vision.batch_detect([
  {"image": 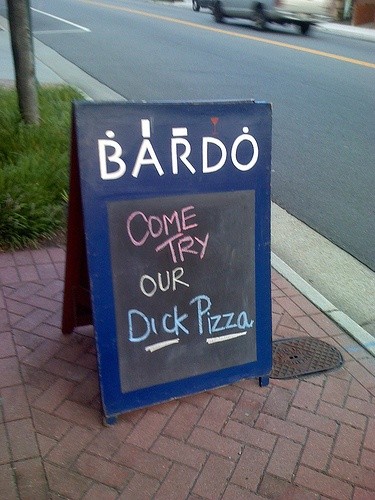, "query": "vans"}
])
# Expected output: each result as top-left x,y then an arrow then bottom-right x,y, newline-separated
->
190,1 -> 331,36
